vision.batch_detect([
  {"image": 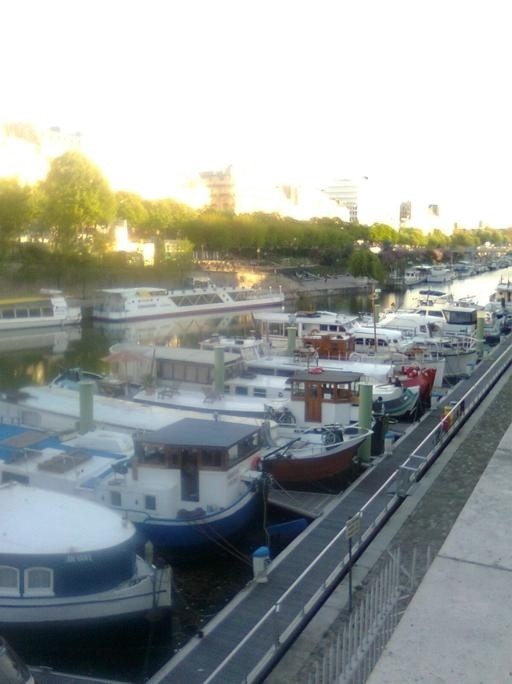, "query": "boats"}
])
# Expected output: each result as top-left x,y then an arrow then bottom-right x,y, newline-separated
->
98,293 -> 486,426
404,256 -> 512,286
92,285 -> 286,332
486,273 -> 512,347
1,382 -> 273,549
0,289 -> 82,330
12,387 -> 373,482
0,479 -> 171,636
